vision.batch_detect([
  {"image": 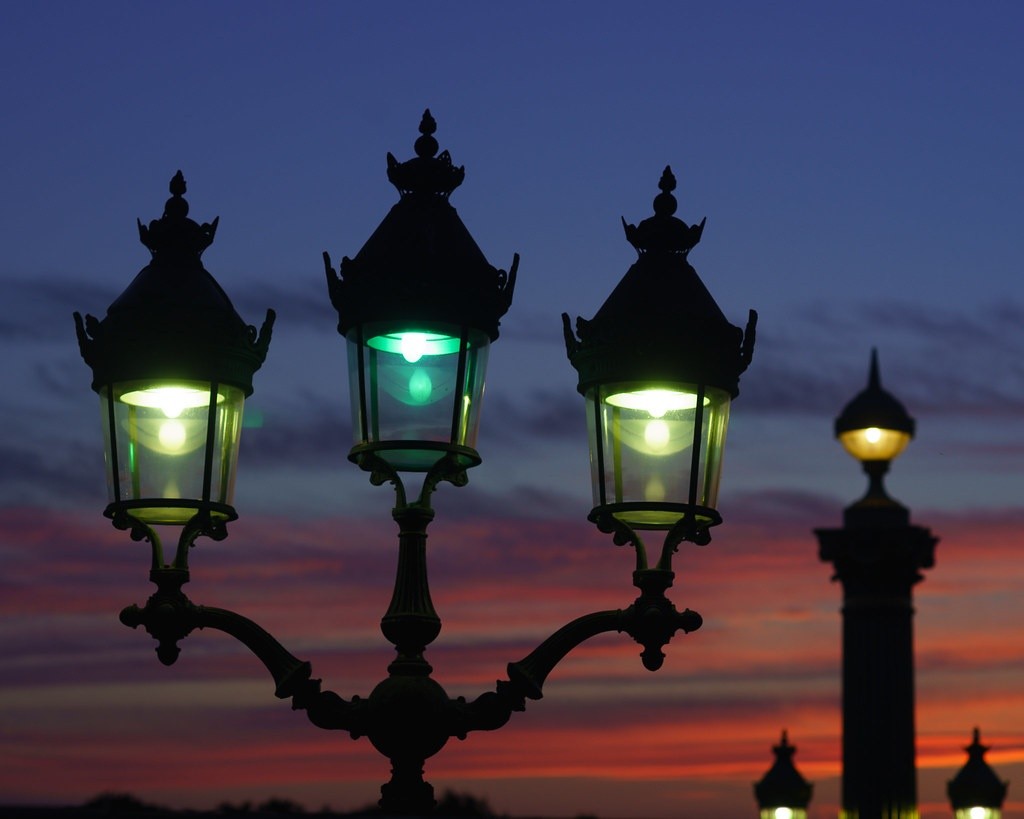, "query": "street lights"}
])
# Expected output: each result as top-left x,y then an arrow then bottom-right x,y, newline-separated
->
753,345 -> 1011,819
74,103 -> 757,819
